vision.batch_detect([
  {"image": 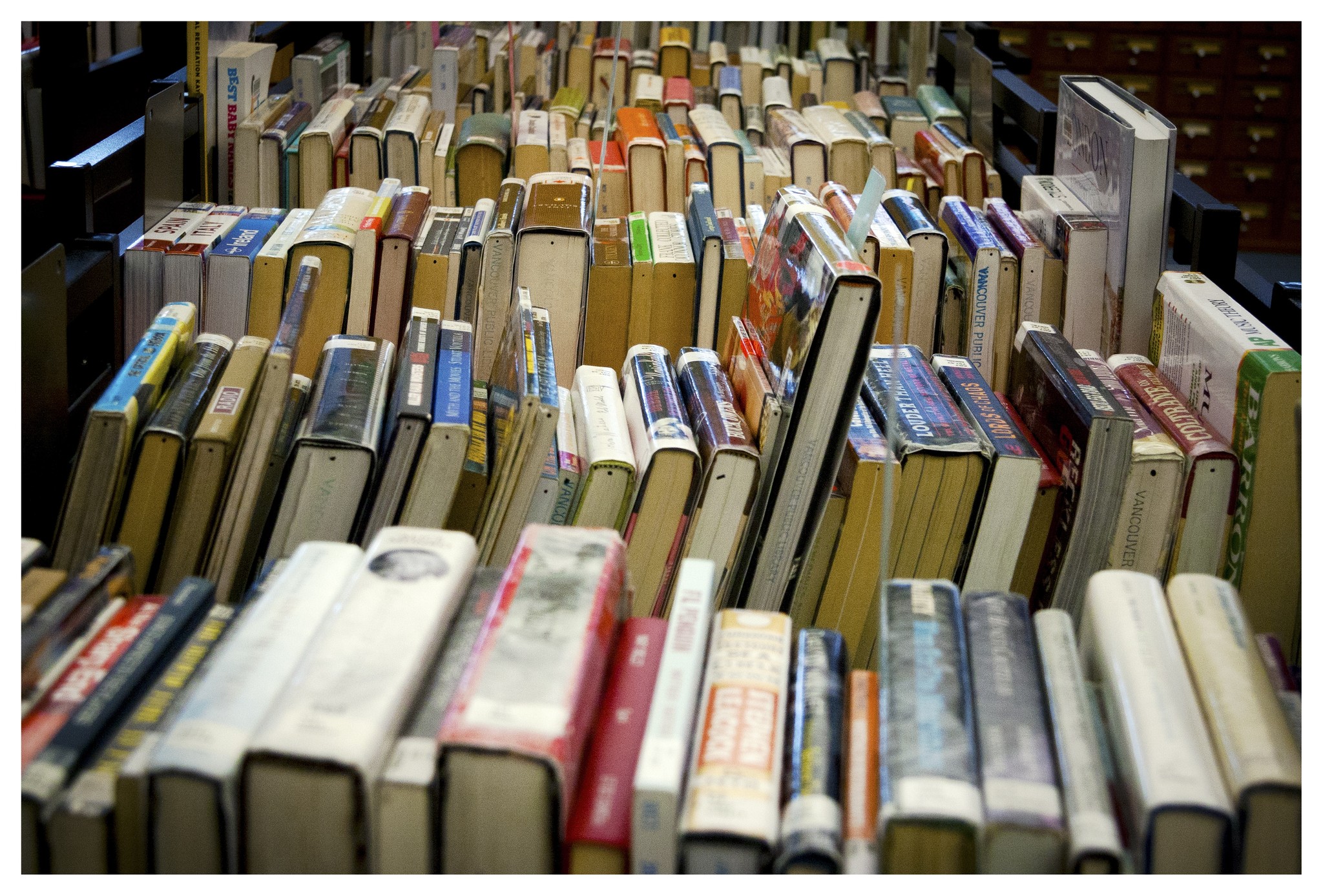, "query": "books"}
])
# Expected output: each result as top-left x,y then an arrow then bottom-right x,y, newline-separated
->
21,24 -> 1303,874
